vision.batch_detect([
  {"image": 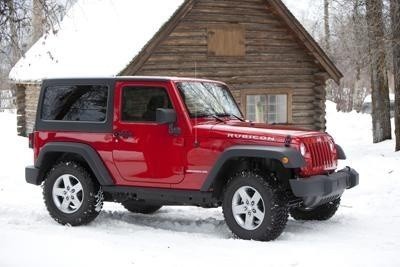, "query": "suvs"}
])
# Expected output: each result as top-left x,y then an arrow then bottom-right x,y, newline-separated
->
24,75 -> 360,242
